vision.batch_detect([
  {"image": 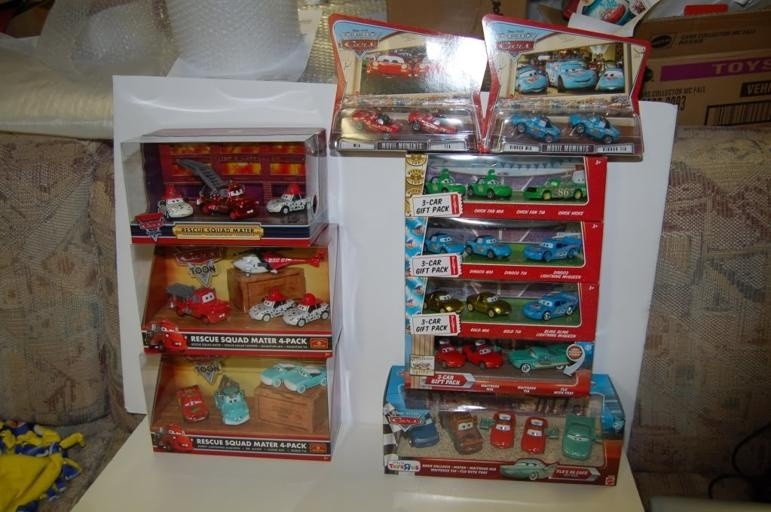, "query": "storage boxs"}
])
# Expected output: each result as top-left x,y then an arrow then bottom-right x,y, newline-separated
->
539,0 -> 771,127
